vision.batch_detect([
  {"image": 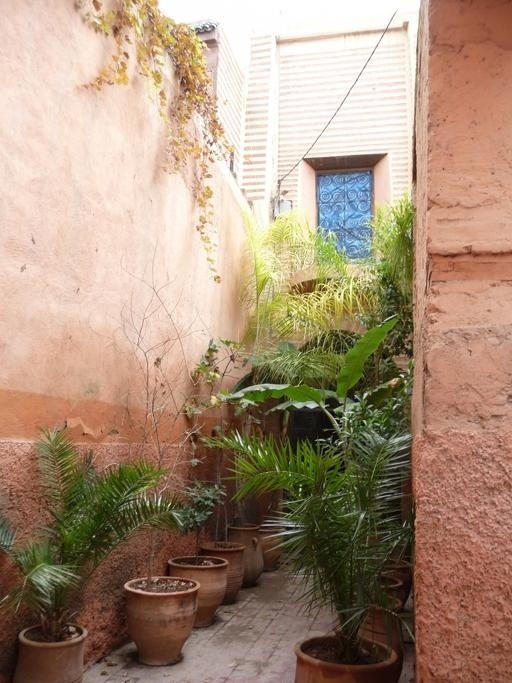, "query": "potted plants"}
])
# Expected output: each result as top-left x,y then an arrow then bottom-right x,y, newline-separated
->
123,314 -> 414,680
3,423 -> 187,680
